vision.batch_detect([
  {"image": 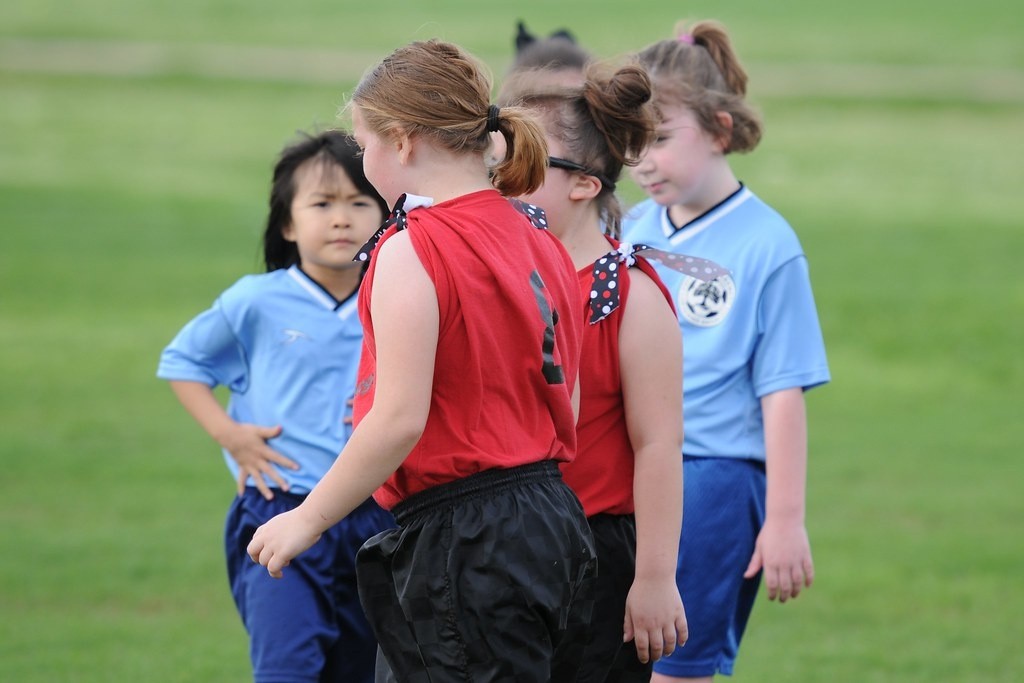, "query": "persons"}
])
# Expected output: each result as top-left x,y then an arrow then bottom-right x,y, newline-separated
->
157,129 -> 398,683
489,65 -> 689,683
510,19 -> 831,683
246,37 -> 598,683
